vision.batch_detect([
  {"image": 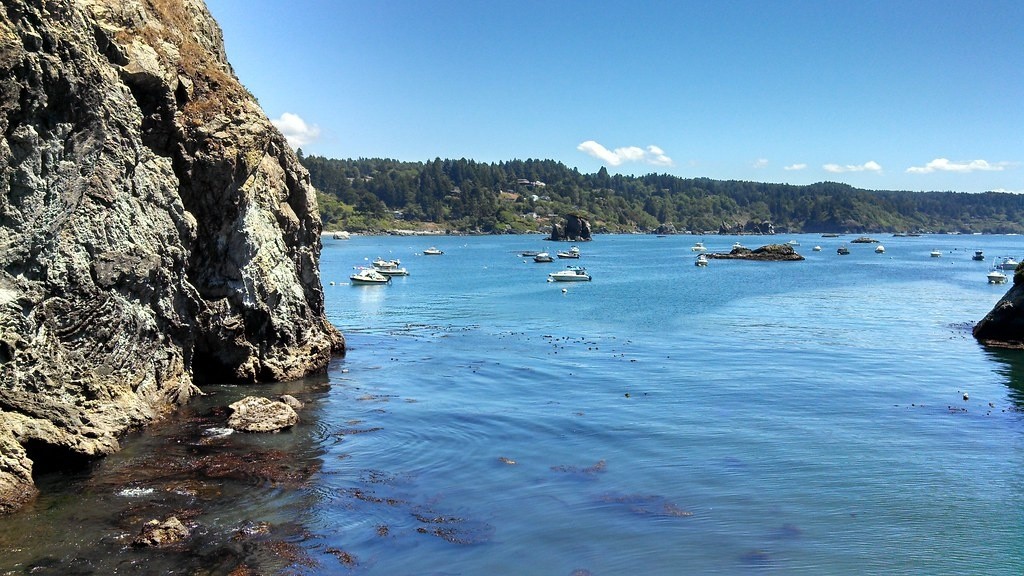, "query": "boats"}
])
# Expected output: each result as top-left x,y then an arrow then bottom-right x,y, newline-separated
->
333,230 -> 351,240
971,250 -> 985,261
986,266 -> 1008,285
372,257 -> 410,276
549,266 -> 593,281
931,252 -> 942,257
423,247 -> 443,255
813,245 -> 822,251
691,243 -> 708,252
732,242 -> 746,250
993,256 -> 1018,270
555,246 -> 581,260
787,240 -> 800,246
349,267 -> 389,286
837,248 -> 850,256
875,246 -> 885,254
533,253 -> 554,263
694,253 -> 709,267
